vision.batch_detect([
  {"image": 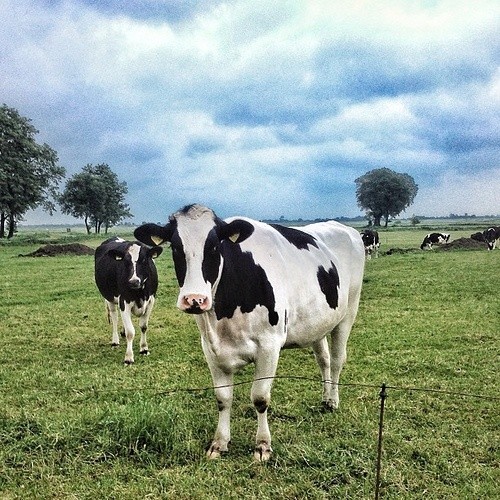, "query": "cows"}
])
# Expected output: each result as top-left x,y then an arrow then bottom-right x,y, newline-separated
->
420,233 -> 451,251
93,234 -> 163,365
133,204 -> 365,461
486,226 -> 500,250
360,230 -> 381,260
